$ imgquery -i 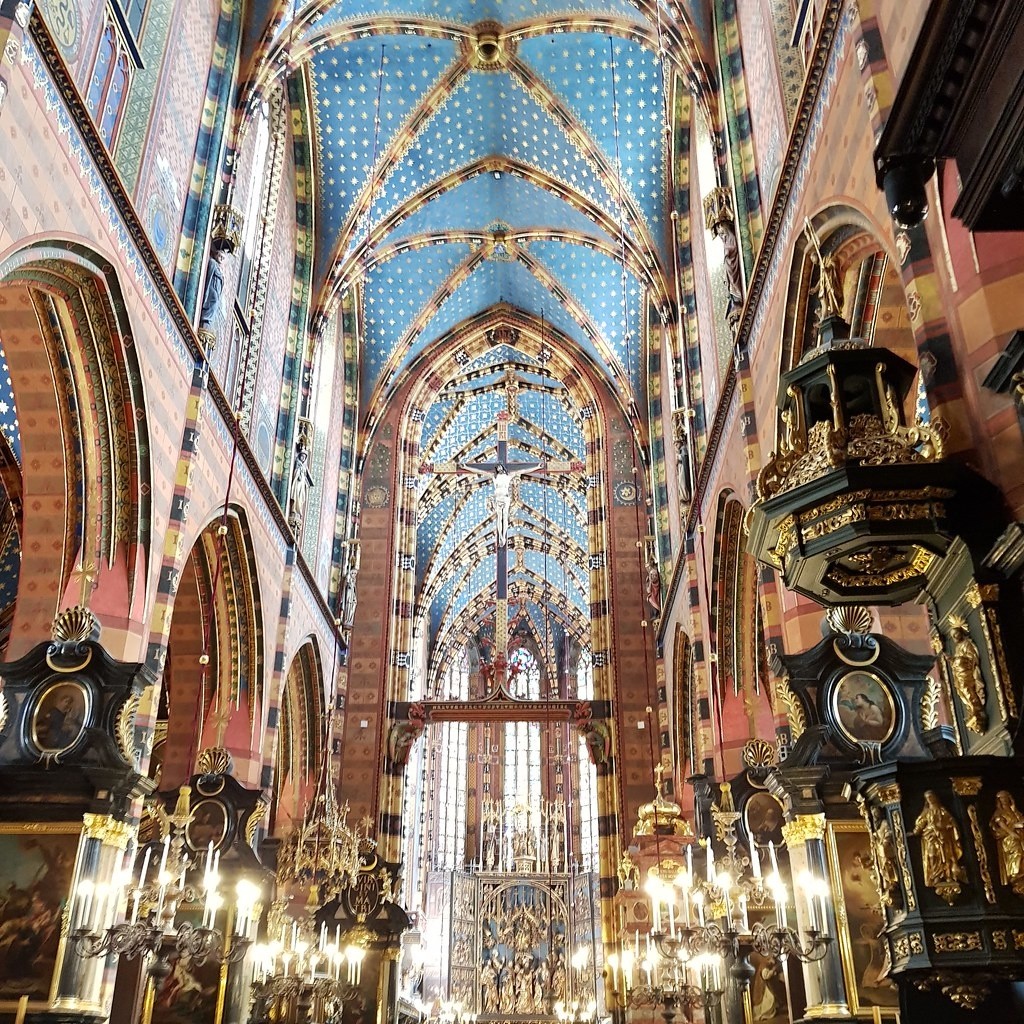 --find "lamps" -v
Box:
[247,41,387,1024]
[71,2,296,988]
[609,0,834,1024]
